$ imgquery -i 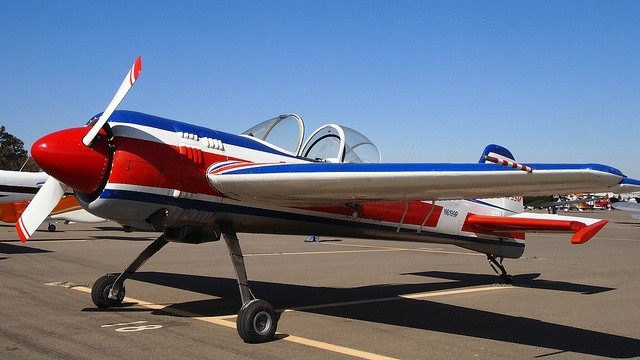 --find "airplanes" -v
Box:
[0,170,113,231]
[0,56,640,344]
